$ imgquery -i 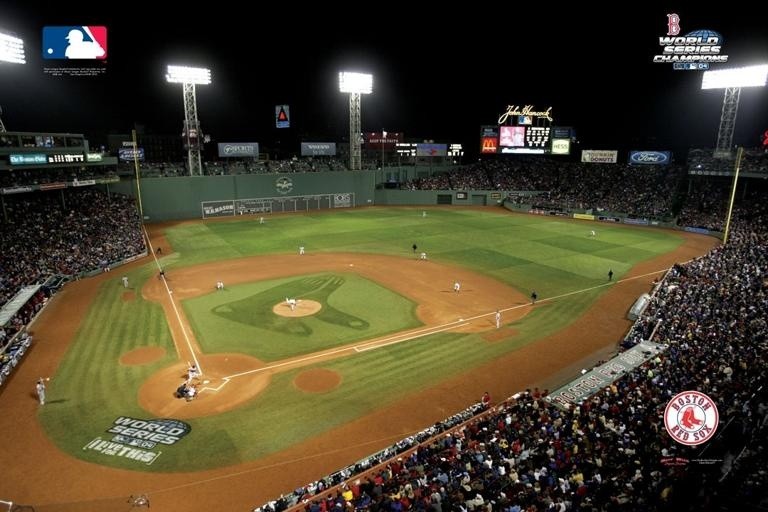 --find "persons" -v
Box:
[247,382,617,511]
[187,364,198,383]
[618,238,767,512]
[260,216,265,225]
[283,296,303,309]
[299,246,309,255]
[141,146,375,176]
[608,267,614,282]
[0,165,146,386]
[36,378,46,406]
[214,279,223,289]
[531,290,538,304]
[495,309,502,329]
[176,383,190,397]
[454,281,460,296]
[383,143,767,238]
[420,251,428,260]
[412,244,418,253]
